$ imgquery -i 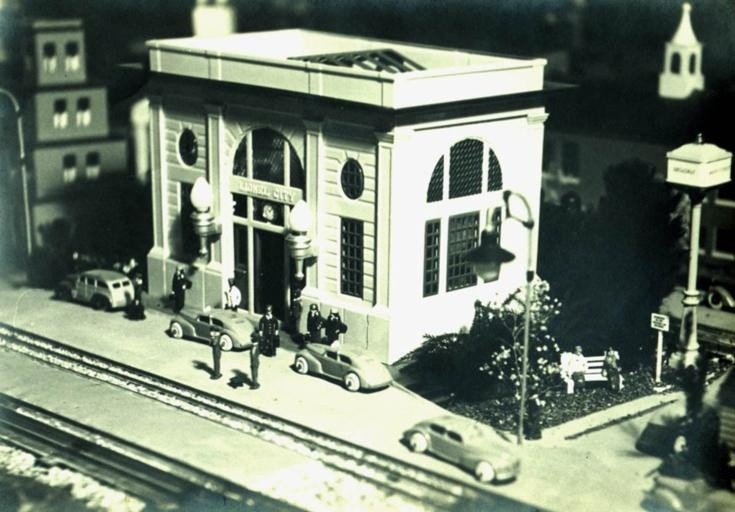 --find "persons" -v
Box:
[208,325,225,379]
[223,278,242,313]
[249,338,260,390]
[171,269,192,314]
[565,344,589,396]
[213,326,225,350]
[325,308,348,346]
[306,303,324,344]
[600,346,622,394]
[258,304,280,358]
[113,256,147,321]
